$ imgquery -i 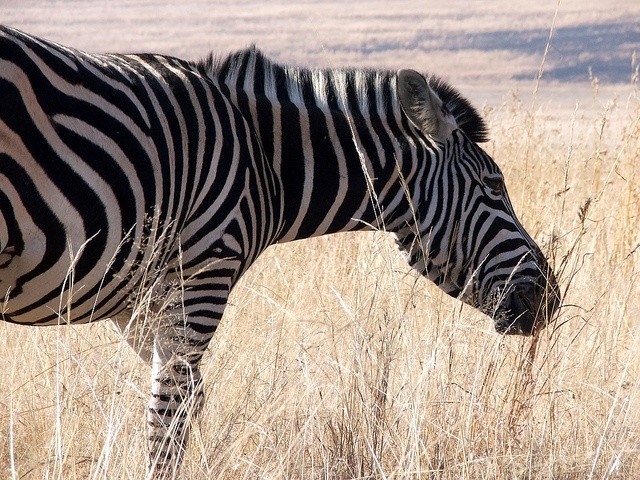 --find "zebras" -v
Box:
[1,23,561,480]
